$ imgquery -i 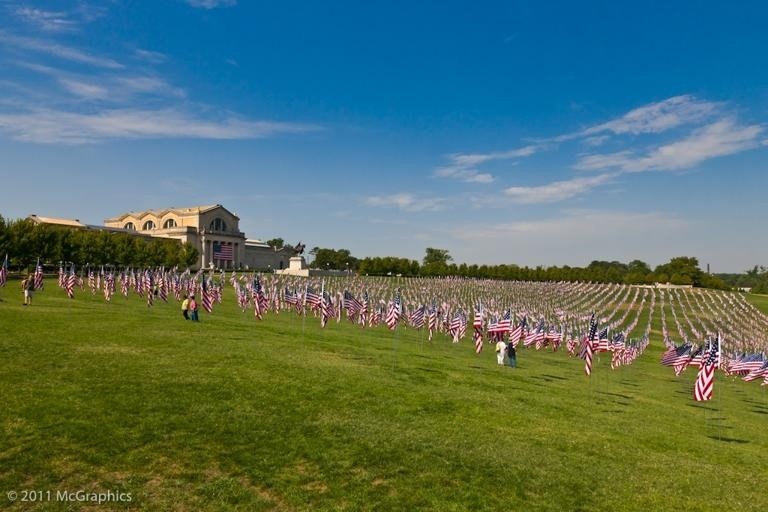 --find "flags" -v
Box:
[214,243,232,261]
